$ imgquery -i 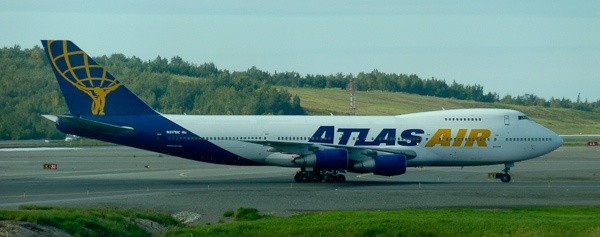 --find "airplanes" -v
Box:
[40,39,565,184]
